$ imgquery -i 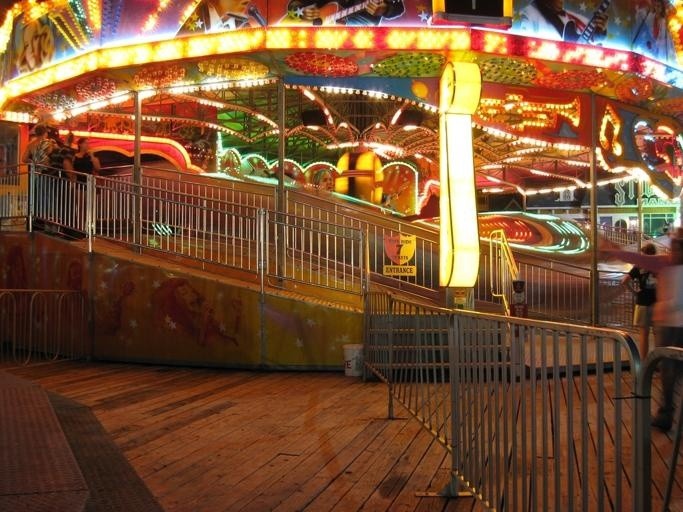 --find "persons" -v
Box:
[506,0,609,47]
[177,1,254,37]
[287,0,404,27]
[313,168,334,197]
[17,19,54,72]
[599,227,683,432]
[621,244,662,372]
[631,0,678,67]
[23,124,100,240]
